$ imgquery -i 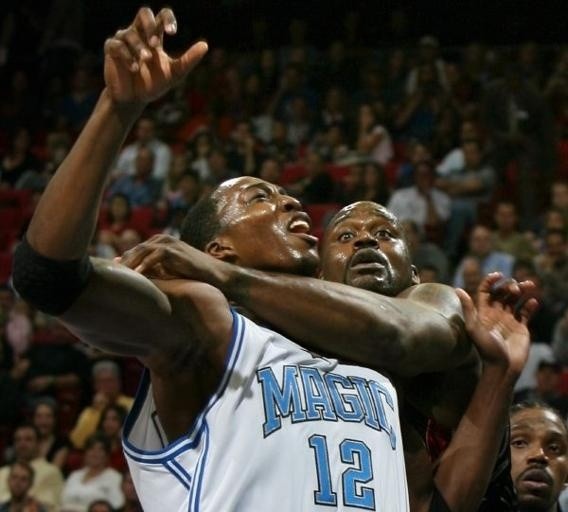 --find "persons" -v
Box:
[0,1,568,512]
